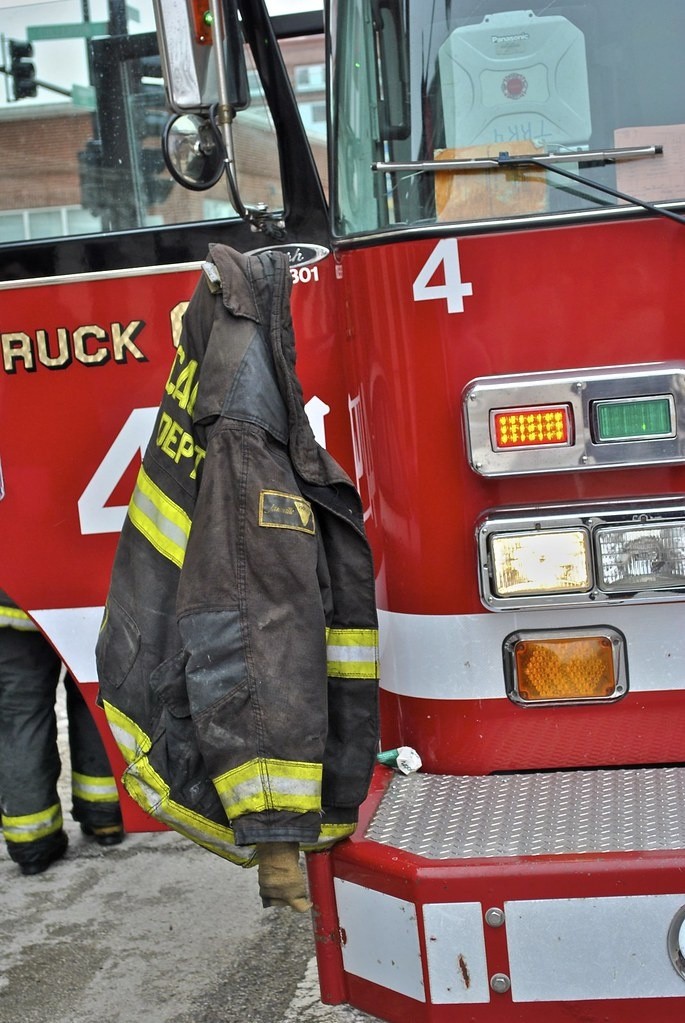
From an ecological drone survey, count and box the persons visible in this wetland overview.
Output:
[0,588,125,875]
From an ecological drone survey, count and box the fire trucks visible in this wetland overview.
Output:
[1,0,685,1023]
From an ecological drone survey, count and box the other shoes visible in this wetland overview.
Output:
[19,831,68,875]
[80,821,122,845]
